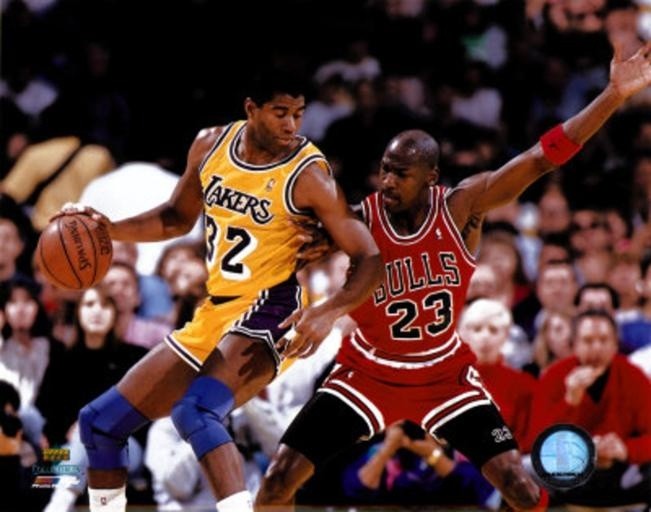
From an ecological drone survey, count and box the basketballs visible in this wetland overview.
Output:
[38,214,112,289]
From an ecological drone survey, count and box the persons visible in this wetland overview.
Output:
[1,0,651,510]
